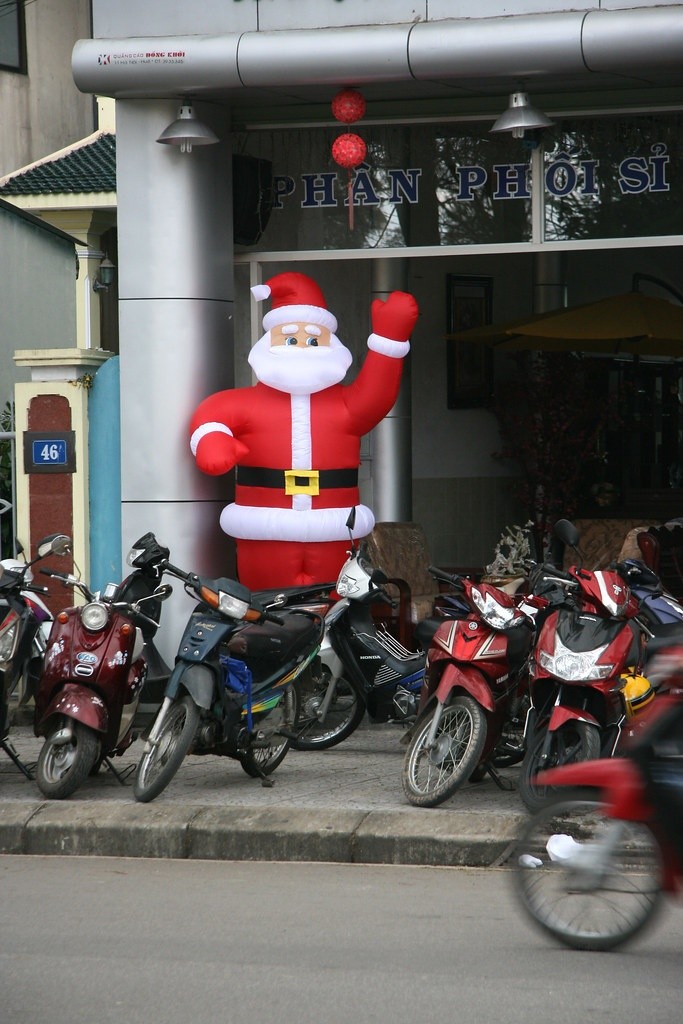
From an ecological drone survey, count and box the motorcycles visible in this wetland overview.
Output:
[0,515,683,958]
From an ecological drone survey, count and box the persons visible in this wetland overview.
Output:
[189,271,420,591]
